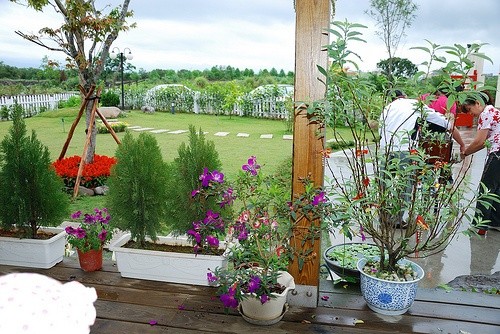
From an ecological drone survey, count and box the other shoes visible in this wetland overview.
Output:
[379,217,411,230]
[477,228,488,236]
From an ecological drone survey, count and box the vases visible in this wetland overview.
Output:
[357,257,424,316]
[239,267,296,319]
[77,247,102,272]
[324,243,387,282]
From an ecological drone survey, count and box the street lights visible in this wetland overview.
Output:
[109,47,133,107]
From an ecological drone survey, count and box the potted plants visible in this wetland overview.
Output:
[0,98,66,269]
[104,124,234,286]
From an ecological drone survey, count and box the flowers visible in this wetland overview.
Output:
[64,207,113,256]
[302,0,500,271]
[189,155,327,307]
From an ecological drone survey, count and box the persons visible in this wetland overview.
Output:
[378,89,465,229]
[459,92,500,235]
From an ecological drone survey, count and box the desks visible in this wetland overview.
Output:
[455,113,473,129]
